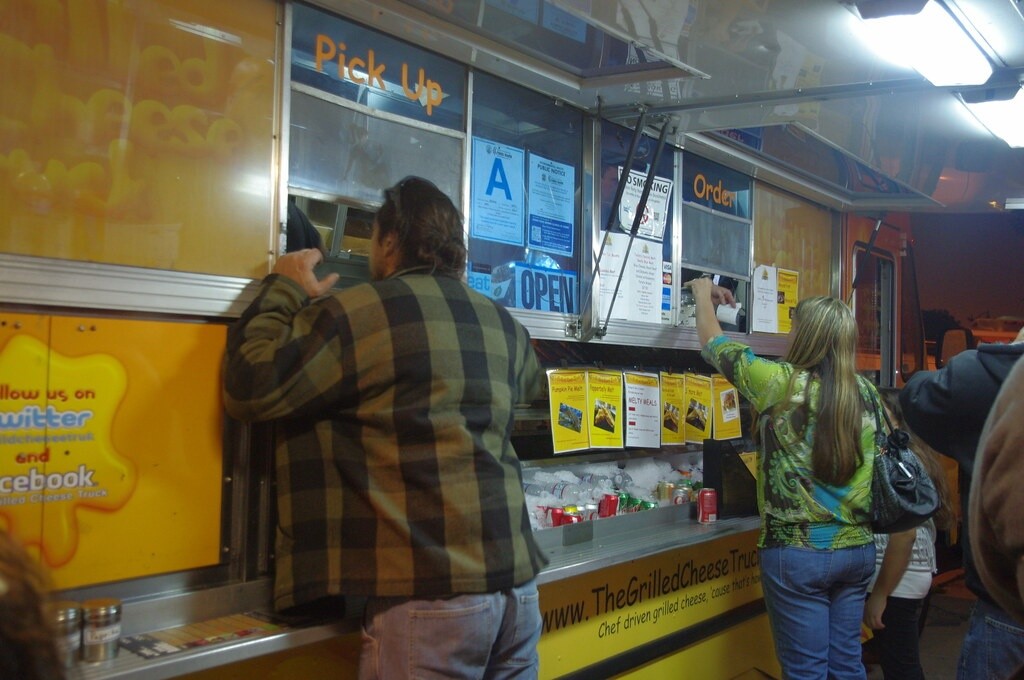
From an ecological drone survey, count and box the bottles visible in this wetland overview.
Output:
[523,471,634,531]
[83,599,121,660]
[47,601,82,668]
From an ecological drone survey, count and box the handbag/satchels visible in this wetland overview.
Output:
[860,375,940,534]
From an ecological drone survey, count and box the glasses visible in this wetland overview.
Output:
[389,181,402,219]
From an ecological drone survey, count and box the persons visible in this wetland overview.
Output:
[221,175,550,680]
[600,157,633,232]
[286,198,329,257]
[684,277,954,680]
[898,323,1024,680]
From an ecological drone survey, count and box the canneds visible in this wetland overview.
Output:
[698,487,717,525]
[533,478,702,527]
